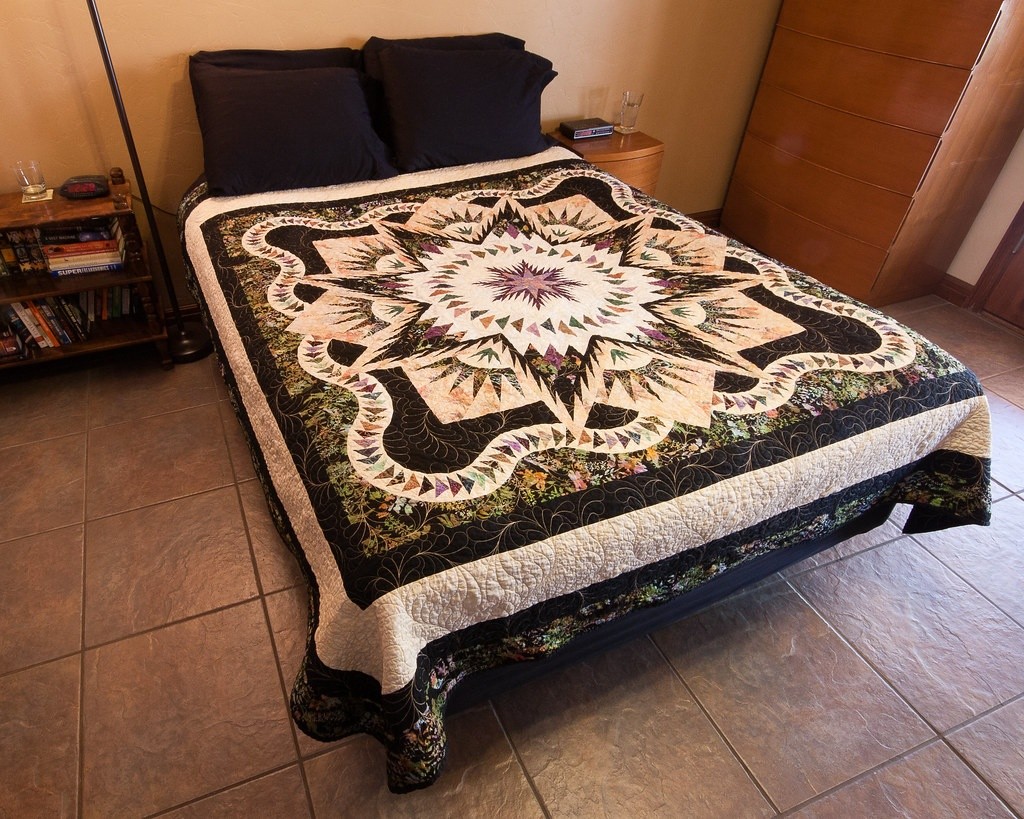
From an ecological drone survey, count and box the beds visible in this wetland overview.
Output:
[172,131,991,798]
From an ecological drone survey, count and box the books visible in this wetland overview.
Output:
[1,215,163,358]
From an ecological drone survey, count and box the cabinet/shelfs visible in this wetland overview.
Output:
[717,0,1024,309]
[0,167,173,383]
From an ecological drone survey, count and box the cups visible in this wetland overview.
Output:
[12,160,48,199]
[620,91,645,132]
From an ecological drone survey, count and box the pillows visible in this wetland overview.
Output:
[193,45,386,145]
[361,32,528,148]
[188,55,402,197]
[381,45,558,174]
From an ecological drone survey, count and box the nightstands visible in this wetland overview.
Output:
[545,123,665,200]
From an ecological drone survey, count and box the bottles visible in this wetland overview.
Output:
[108,167,132,210]
